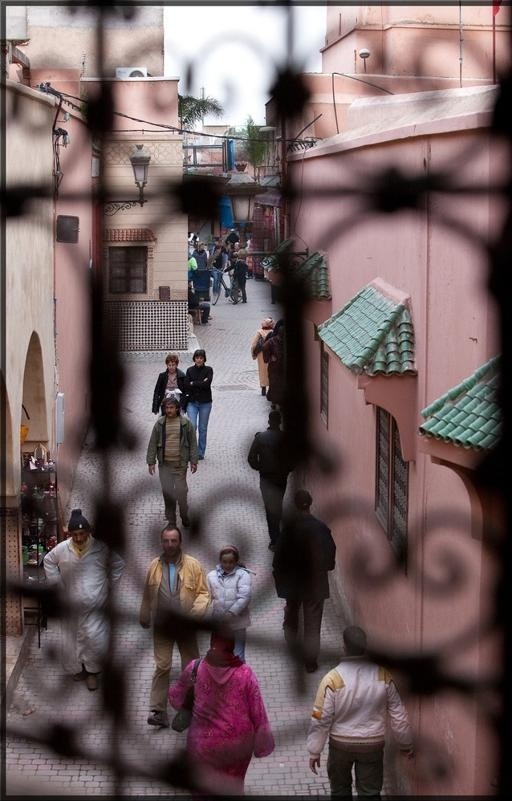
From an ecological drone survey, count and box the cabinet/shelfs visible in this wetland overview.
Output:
[21,450,62,648]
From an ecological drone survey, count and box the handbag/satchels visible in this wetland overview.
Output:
[253,332,264,356]
[169,658,200,735]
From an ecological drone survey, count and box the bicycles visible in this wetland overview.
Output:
[212,267,243,306]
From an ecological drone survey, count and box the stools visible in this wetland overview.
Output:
[188,308,204,325]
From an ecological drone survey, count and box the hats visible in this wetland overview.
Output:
[68,508,90,532]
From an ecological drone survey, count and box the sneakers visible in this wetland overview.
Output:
[198,455,205,460]
[234,300,247,304]
[201,318,209,324]
[304,657,319,675]
[267,541,279,552]
[67,670,99,692]
[181,517,191,527]
[261,387,267,395]
[147,711,171,729]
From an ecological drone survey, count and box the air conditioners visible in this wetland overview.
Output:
[116,65,150,79]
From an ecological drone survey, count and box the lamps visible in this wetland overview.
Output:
[95,142,153,217]
[357,46,371,74]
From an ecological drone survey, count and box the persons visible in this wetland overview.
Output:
[184,350,213,460]
[152,355,188,419]
[206,546,251,663]
[273,490,337,671]
[188,228,247,326]
[248,411,294,551]
[139,529,211,728]
[251,317,284,410]
[147,398,198,527]
[169,627,276,801]
[308,627,415,801]
[44,515,126,690]
